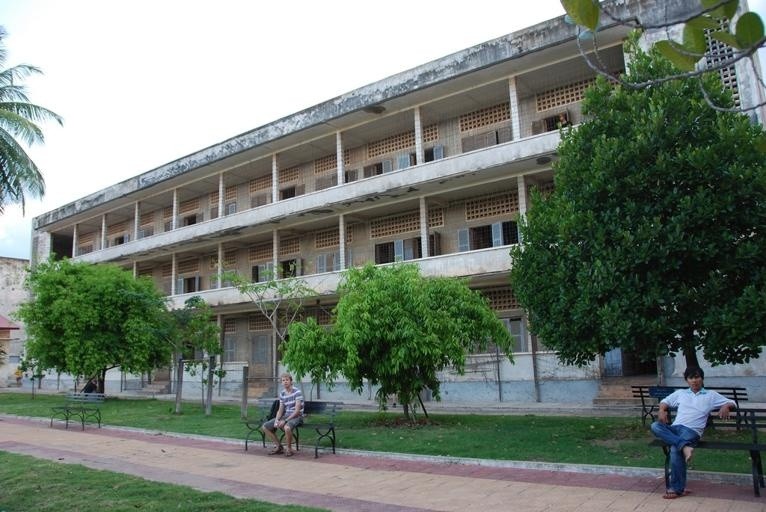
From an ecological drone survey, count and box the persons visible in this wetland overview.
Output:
[84,379,97,396]
[650,365,737,499]
[555,111,572,130]
[262,374,304,456]
[15,365,23,386]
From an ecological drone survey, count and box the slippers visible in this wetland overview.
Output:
[662,490,678,499]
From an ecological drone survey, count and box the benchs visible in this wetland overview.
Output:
[240,400,344,458]
[631,386,766,497]
[47,393,106,431]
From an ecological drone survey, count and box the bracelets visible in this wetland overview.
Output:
[284,418,287,423]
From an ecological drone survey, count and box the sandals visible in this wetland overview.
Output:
[272,445,283,455]
[286,452,293,457]
